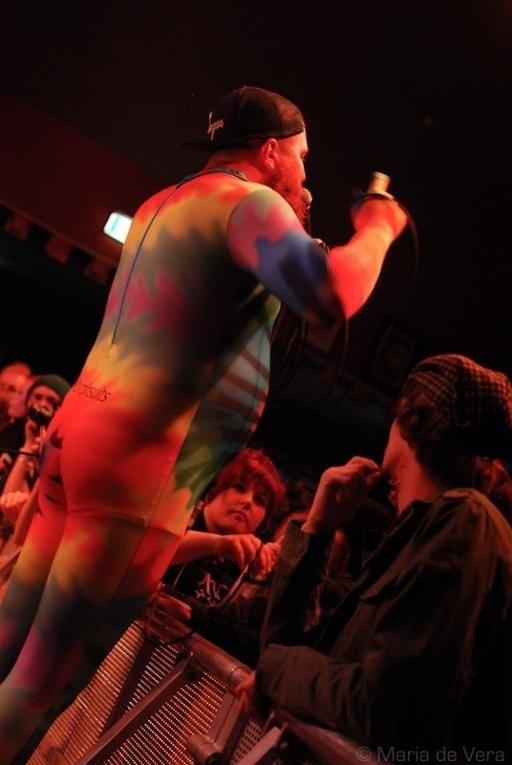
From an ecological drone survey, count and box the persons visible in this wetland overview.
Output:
[0,84,411,765]
[2,355,511,765]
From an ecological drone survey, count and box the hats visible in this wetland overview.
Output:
[404,352,511,443]
[178,85,305,148]
[25,373,70,407]
